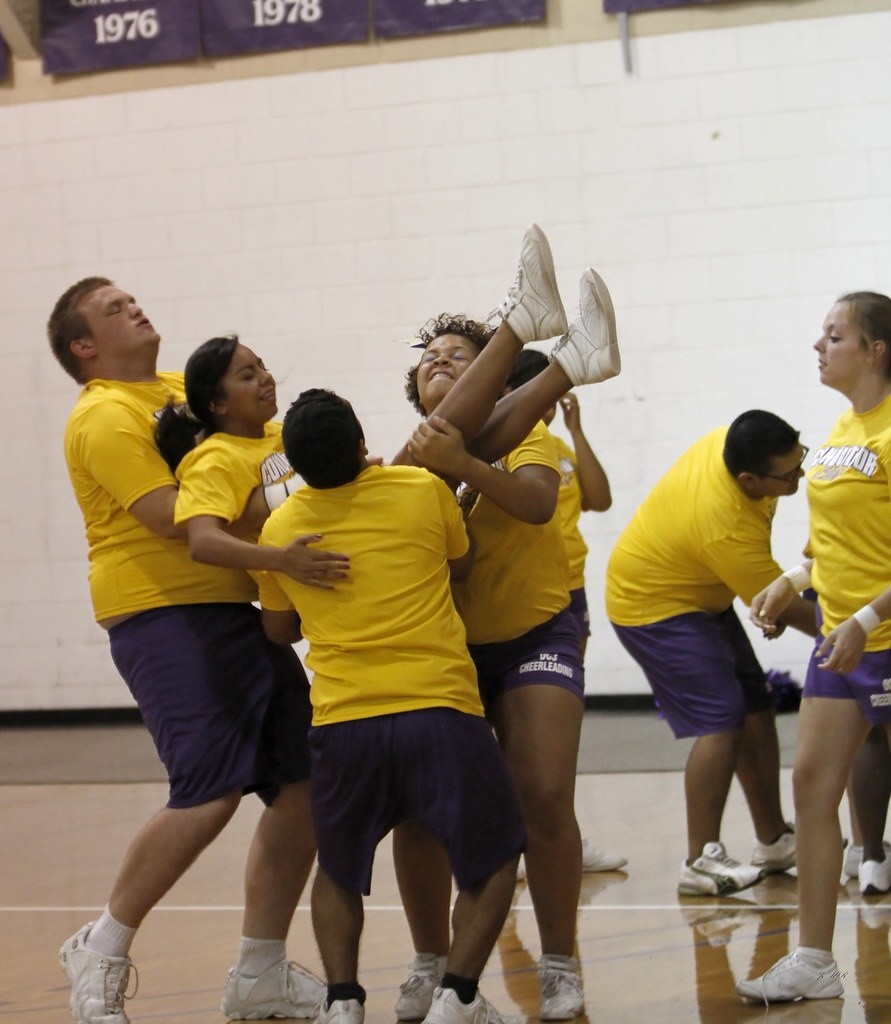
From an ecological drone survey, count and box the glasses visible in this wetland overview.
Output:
[767,444,810,483]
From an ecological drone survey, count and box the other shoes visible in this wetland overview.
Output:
[495,881,526,938]
[676,841,766,896]
[843,838,891,877]
[676,896,759,947]
[750,820,849,875]
[860,894,891,930]
[581,836,628,872]
[858,846,891,896]
[579,870,628,906]
[752,871,851,907]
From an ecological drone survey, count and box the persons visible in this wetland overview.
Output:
[48,225,632,1023]
[737,292,891,1000]
[606,409,816,896]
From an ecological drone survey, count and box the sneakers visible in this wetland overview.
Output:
[538,940,586,1020]
[219,957,325,1018]
[486,222,570,344]
[733,951,845,1008]
[393,951,448,1021]
[307,993,366,1024]
[58,921,140,1024]
[421,987,508,1024]
[547,266,622,386]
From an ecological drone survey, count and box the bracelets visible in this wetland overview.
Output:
[782,564,811,594]
[851,605,879,634]
